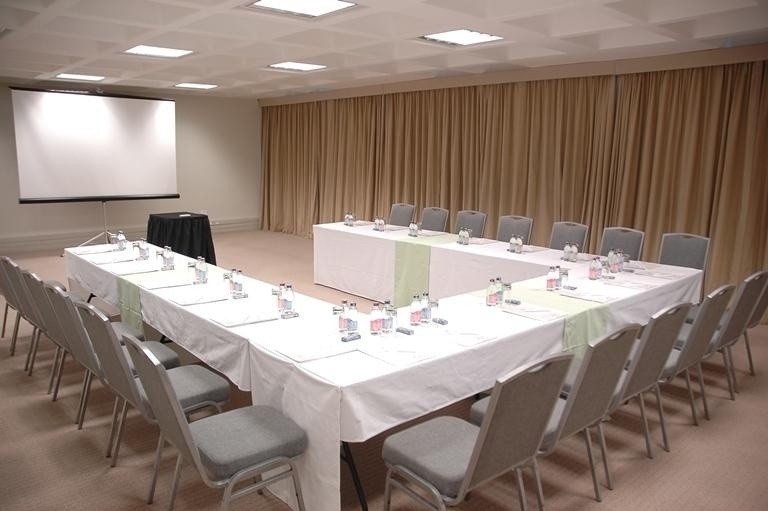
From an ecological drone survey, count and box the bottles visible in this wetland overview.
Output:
[117,231,126,250]
[338,301,358,336]
[563,241,577,262]
[588,248,624,281]
[344,212,354,226]
[410,292,431,325]
[373,216,385,232]
[194,257,206,280]
[228,268,243,293]
[370,299,393,335]
[408,221,418,236]
[161,246,173,265]
[486,276,504,307]
[508,234,522,252]
[277,285,292,311]
[458,227,469,245]
[138,238,150,258]
[544,265,562,292]
